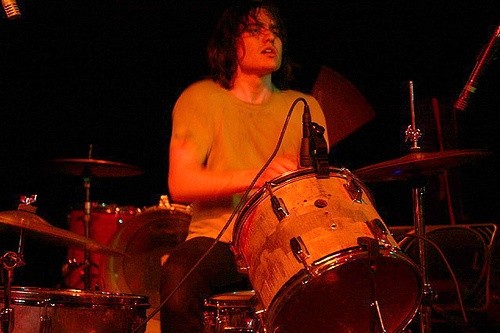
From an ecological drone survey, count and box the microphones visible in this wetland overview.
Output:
[454,26,500,111]
[0,0,22,21]
[300,105,313,166]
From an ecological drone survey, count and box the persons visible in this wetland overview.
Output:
[160,0,330,333]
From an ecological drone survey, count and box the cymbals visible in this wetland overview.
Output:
[353,149,493,182]
[55,159,139,178]
[0,211,131,258]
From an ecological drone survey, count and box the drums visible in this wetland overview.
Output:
[203,291,258,330]
[65,207,160,333]
[232,167,424,333]
[111,205,193,320]
[0,286,149,333]
